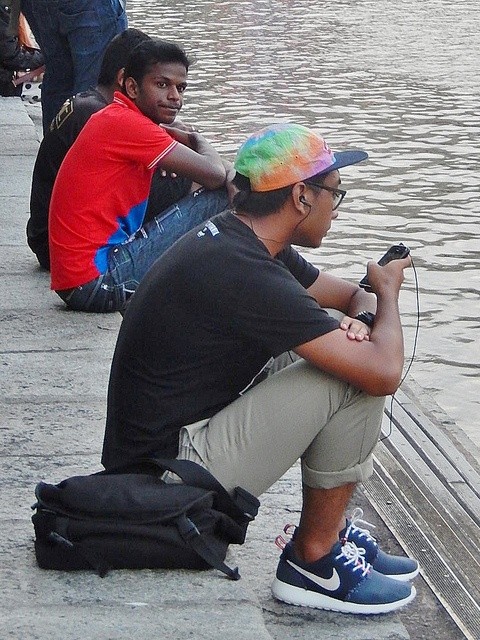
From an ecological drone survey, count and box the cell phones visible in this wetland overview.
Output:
[358,244,410,289]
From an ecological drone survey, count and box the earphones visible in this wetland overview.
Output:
[233,193,422,443]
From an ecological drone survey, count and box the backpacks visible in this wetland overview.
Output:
[31,457,262,579]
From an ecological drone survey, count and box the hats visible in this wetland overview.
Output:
[227,124,368,193]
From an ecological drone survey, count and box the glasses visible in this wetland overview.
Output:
[309,181,346,211]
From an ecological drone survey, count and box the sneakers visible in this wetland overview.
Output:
[284,507,419,582]
[272,536,416,613]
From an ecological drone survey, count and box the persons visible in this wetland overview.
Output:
[48,38,240,313]
[101,123,421,614]
[27,29,195,271]
[20,0,126,140]
